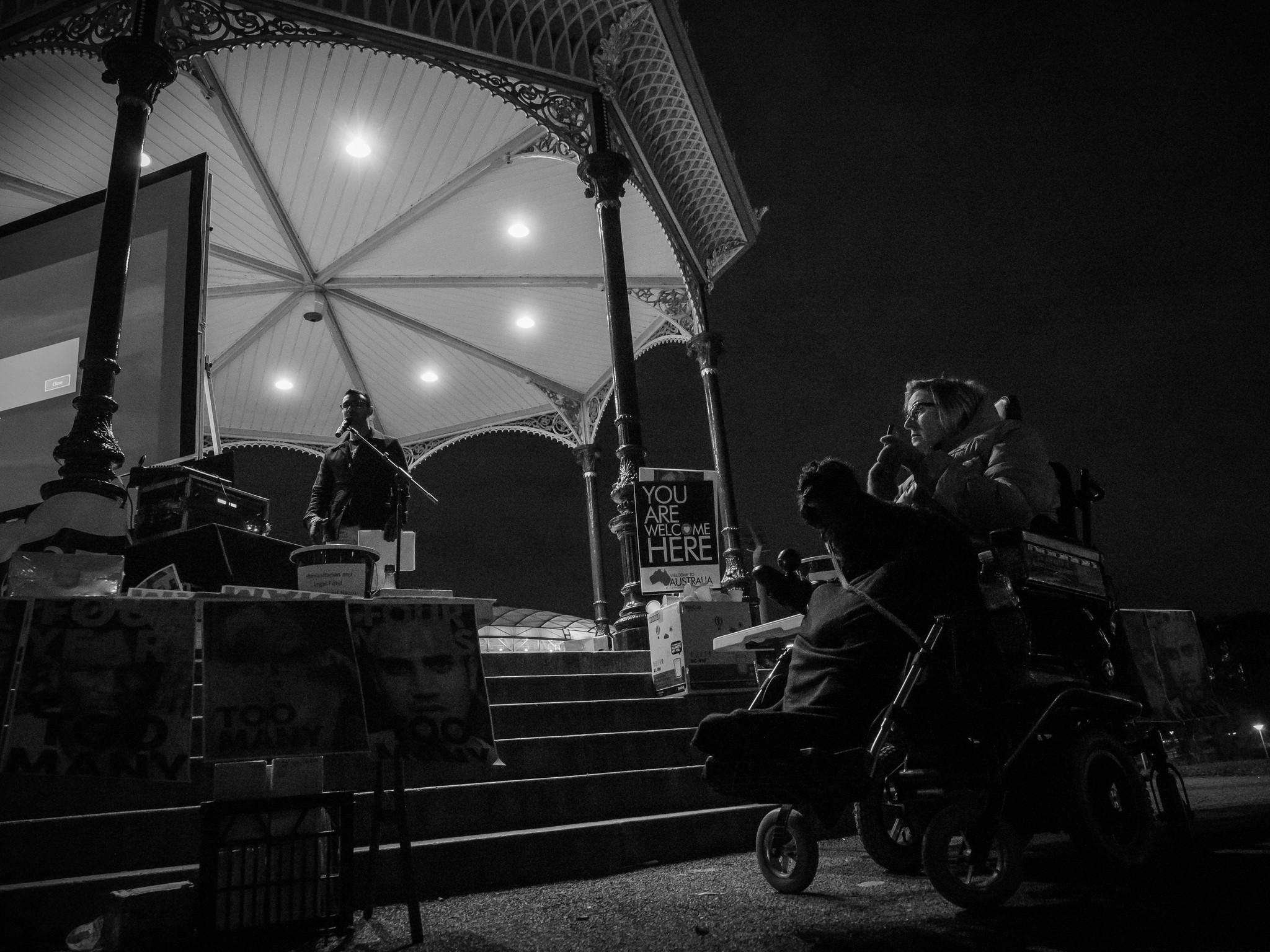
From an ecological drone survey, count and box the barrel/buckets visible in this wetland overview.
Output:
[289,543,381,599]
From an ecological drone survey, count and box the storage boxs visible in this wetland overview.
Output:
[1020,530,1108,602]
[134,473,270,545]
[6,552,125,598]
[647,602,760,698]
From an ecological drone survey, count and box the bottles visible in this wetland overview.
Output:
[382,563,397,589]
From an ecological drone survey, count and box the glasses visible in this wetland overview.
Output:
[908,402,935,418]
[339,401,368,409]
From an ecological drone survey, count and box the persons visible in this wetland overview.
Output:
[1147,613,1227,719]
[302,388,411,545]
[690,377,1063,801]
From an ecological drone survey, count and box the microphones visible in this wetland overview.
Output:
[335,417,353,438]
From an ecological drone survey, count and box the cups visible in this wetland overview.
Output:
[645,583,743,614]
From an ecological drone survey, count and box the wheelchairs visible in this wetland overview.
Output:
[702,464,1205,908]
